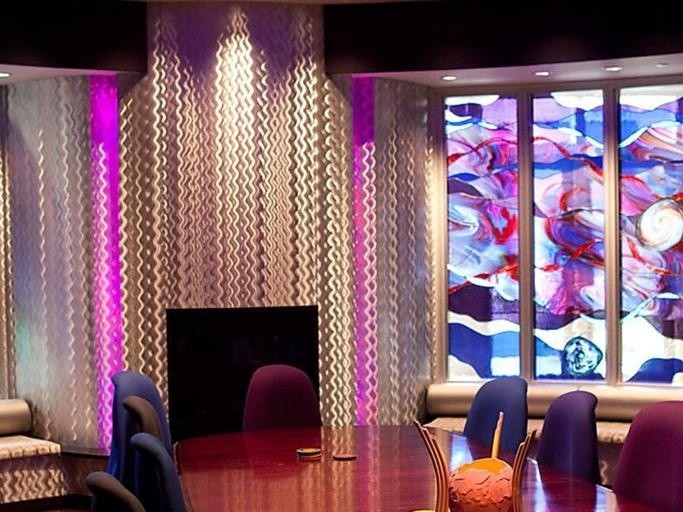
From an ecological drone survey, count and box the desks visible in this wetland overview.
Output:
[176,425,674,512]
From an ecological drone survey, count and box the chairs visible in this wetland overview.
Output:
[242,364,323,431]
[85,370,188,512]
[463,376,683,512]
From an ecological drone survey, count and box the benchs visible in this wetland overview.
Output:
[418,382,682,488]
[0,398,68,503]
